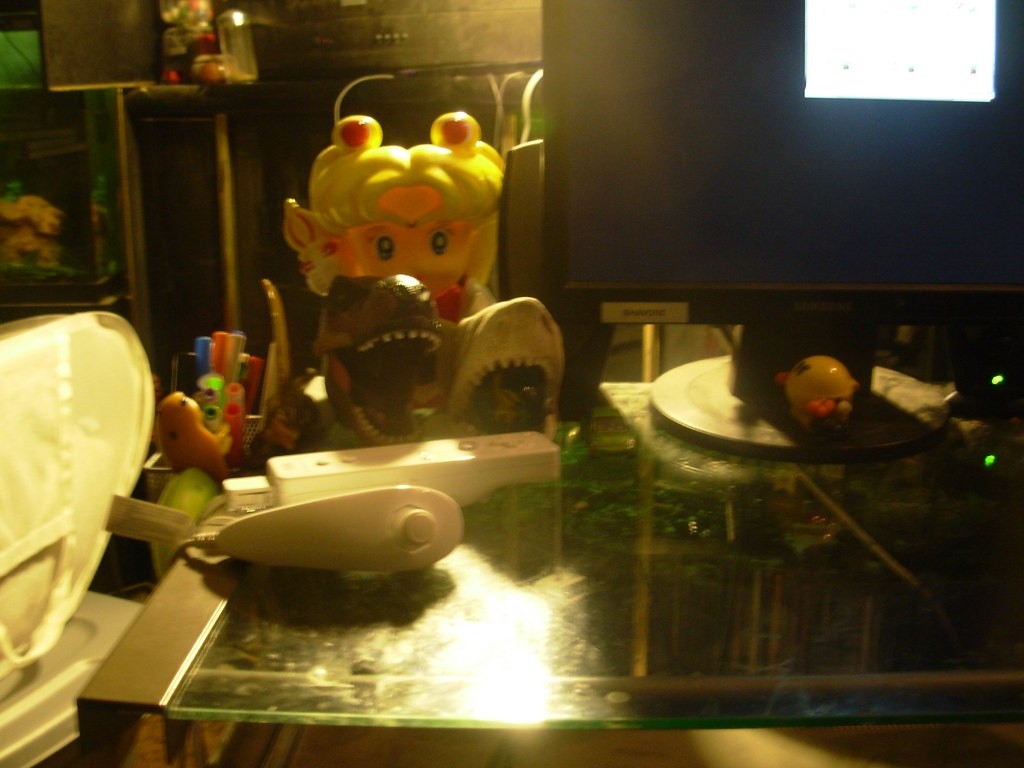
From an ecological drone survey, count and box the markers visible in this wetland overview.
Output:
[170,328,267,468]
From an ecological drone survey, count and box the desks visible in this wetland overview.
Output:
[75,381,1023,763]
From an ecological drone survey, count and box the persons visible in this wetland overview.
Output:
[284,113,507,323]
